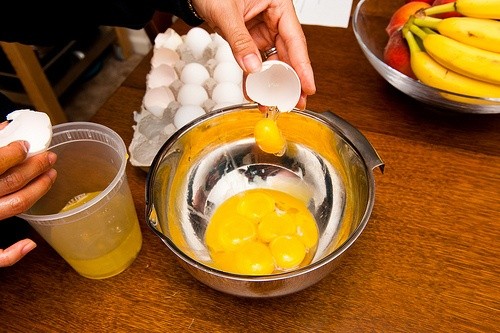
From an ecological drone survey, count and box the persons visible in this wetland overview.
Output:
[0,0,319,268]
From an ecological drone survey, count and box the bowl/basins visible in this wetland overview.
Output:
[145,102,385,299]
[352,0,500,114]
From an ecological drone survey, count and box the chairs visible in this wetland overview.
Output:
[1,26,135,126]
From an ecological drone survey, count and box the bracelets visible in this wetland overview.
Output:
[186,0,204,23]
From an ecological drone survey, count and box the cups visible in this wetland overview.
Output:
[16,122,143,279]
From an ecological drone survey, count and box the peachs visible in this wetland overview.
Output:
[385,0,463,78]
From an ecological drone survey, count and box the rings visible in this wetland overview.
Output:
[263,46,278,59]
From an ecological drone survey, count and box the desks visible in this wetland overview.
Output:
[0,19,500,333]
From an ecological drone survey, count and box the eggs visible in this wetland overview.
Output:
[254,107,287,157]
[204,191,319,275]
[144,27,245,131]
[45,190,142,282]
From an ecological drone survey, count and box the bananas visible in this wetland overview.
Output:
[400,0,500,105]
